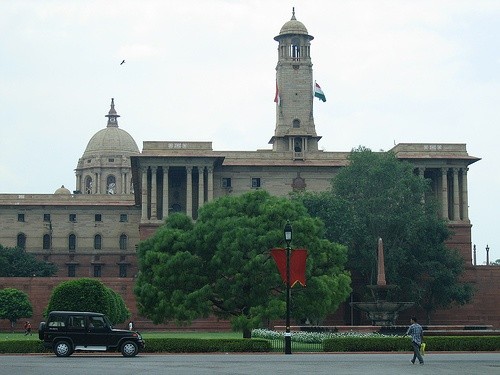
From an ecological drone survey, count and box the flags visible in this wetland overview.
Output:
[274,81,279,103]
[315,83,326,102]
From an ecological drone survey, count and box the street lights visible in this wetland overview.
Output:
[485,245,490,265]
[283,219,293,355]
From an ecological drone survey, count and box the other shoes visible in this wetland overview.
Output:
[419,362,424,366]
[410,360,414,365]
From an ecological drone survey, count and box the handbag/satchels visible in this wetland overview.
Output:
[420,342,426,356]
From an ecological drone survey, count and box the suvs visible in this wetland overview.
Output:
[38,310,145,358]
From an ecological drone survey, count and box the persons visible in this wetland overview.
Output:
[90,319,94,327]
[24,321,32,336]
[129,321,135,330]
[403,317,424,365]
